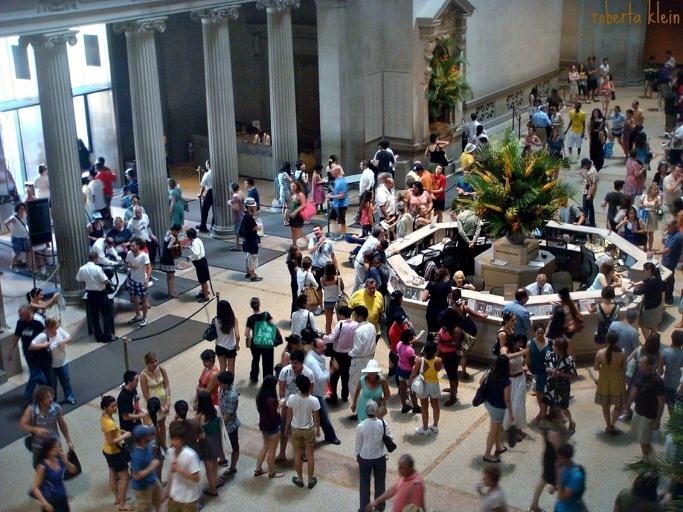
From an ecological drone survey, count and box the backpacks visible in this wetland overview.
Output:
[598,304,618,338]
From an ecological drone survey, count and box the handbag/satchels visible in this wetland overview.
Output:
[637,231,647,244]
[323,344,333,357]
[456,331,477,357]
[25,435,35,451]
[35,347,52,371]
[438,151,449,166]
[294,172,306,190]
[411,375,423,395]
[253,321,278,348]
[203,325,217,340]
[65,449,81,480]
[301,284,322,307]
[335,295,349,308]
[300,202,317,220]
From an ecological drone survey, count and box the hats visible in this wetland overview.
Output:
[244,197,257,207]
[401,504,424,512]
[134,424,155,438]
[580,158,588,166]
[361,360,382,373]
[92,212,102,219]
[464,143,476,153]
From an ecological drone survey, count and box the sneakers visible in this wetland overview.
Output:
[231,247,242,251]
[197,292,210,302]
[246,272,263,281]
[128,313,148,327]
[169,291,180,298]
[584,99,600,104]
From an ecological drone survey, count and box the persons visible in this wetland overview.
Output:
[0,47,682,511]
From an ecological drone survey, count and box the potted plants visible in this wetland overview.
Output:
[451,126,579,244]
[427,35,472,138]
